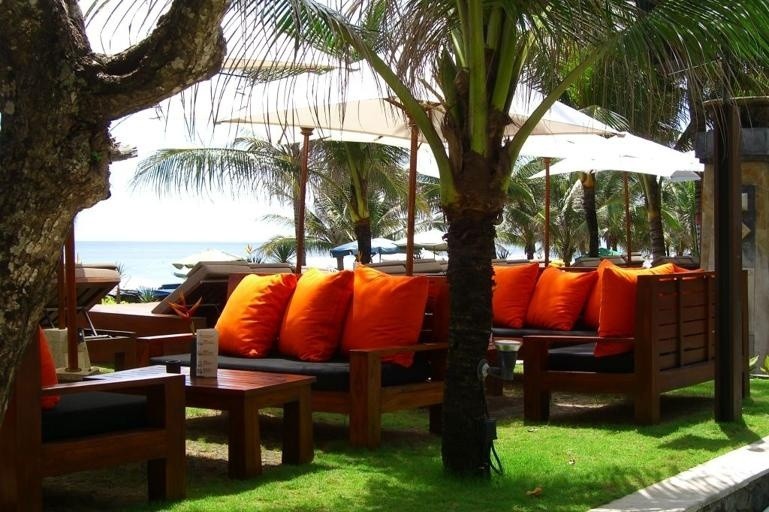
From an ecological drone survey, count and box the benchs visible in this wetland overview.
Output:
[519,264,753,428]
[2,320,188,512]
[479,258,684,348]
[117,258,449,447]
[89,259,300,340]
[41,259,122,330]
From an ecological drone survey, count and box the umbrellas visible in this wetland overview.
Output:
[64,0,360,372]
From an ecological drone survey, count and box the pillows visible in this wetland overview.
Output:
[213,273,295,356]
[338,255,430,370]
[33,320,62,408]
[487,255,708,357]
[277,265,355,363]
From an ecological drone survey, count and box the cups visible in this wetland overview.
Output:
[166,359,180,373]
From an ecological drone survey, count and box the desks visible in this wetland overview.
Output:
[85,362,315,480]
